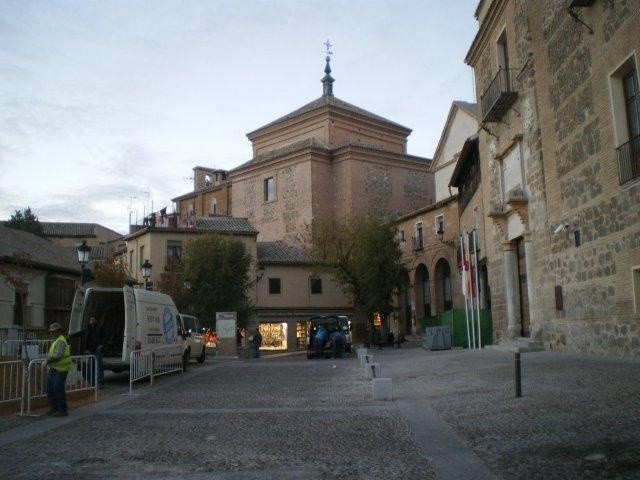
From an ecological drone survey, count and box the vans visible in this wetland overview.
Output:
[306,315,353,359]
[180,314,206,363]
[67,286,192,387]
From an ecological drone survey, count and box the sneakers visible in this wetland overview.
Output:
[54,411,68,416]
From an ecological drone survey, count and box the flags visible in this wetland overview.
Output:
[460,235,477,298]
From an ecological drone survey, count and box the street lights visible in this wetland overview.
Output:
[142,259,152,290]
[77,240,90,286]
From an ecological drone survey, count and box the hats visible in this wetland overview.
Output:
[48,322,63,331]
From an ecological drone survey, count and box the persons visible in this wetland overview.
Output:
[315,327,345,359]
[365,330,404,350]
[84,314,105,389]
[252,327,261,358]
[43,322,72,416]
